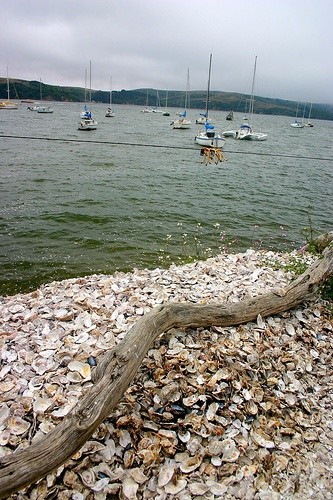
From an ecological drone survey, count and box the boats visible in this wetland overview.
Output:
[225,107,234,120]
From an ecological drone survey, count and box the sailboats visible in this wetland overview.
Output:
[76,59,98,132]
[290,100,315,128]
[139,65,216,130]
[0,66,55,114]
[104,75,115,117]
[243,99,249,120]
[193,53,228,149]
[222,55,268,142]
